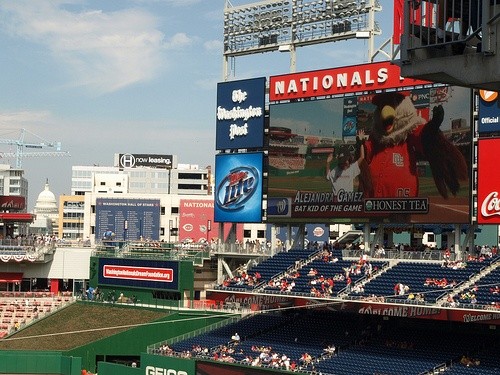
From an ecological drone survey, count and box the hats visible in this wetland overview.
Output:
[337,150,350,157]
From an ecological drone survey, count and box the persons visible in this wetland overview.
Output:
[0,233,500,375]
[323,89,468,225]
